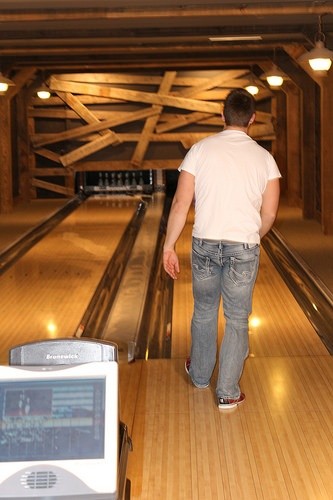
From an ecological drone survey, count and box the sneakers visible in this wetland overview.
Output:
[217,392,247,410]
[184,355,210,389]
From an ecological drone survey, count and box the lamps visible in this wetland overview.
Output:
[0,72,16,92]
[243,76,260,95]
[297,14,333,71]
[258,47,287,86]
[34,82,52,100]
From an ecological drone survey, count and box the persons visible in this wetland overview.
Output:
[162,87,281,408]
